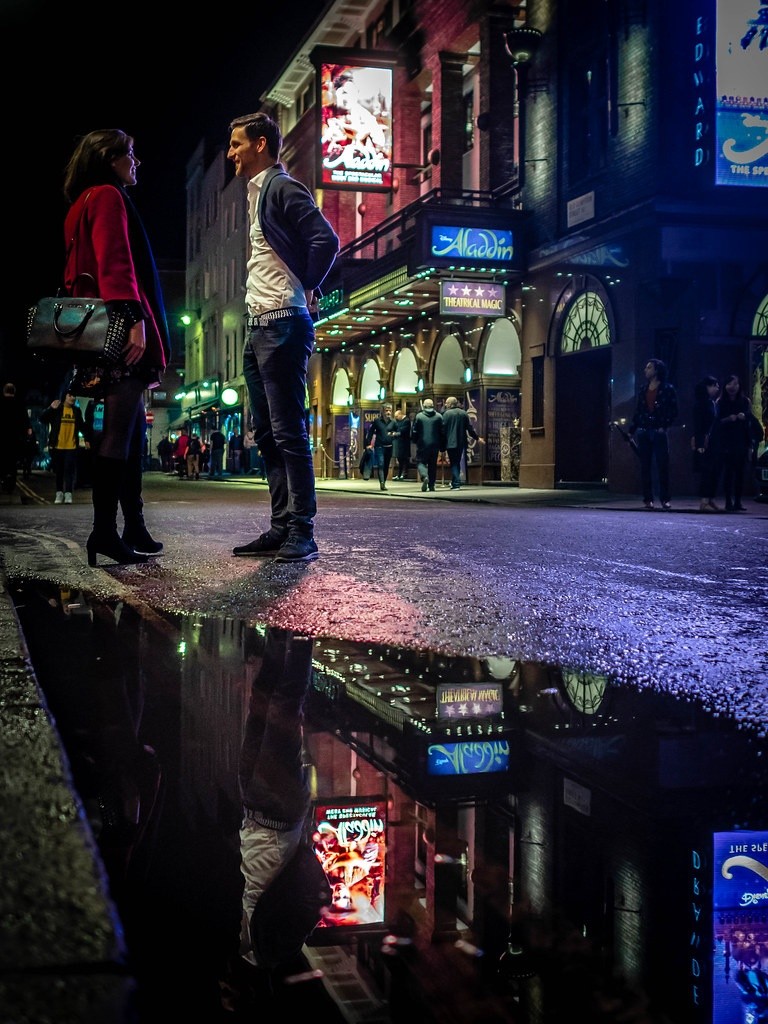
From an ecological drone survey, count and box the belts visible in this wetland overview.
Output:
[244,307,310,327]
[241,805,307,832]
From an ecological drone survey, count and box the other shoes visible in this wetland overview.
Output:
[645,504,654,509]
[663,504,671,509]
[725,503,737,511]
[709,502,726,512]
[63,492,73,504]
[700,503,715,512]
[165,468,241,482]
[54,491,64,505]
[378,469,462,493]
[734,504,747,511]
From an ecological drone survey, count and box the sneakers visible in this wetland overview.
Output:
[273,535,319,562]
[233,531,285,557]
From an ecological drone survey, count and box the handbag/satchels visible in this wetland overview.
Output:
[746,410,765,442]
[26,272,128,367]
[691,435,709,450]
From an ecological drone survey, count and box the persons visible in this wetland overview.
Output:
[82,592,163,833]
[365,401,402,490]
[0,380,104,505]
[719,376,751,512]
[694,374,722,513]
[244,425,258,448]
[391,409,411,481]
[225,112,340,564]
[441,395,486,490]
[411,397,443,492]
[626,358,681,509]
[228,425,243,476]
[157,426,225,482]
[228,628,334,991]
[62,128,164,566]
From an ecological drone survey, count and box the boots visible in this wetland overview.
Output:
[86,455,149,566]
[119,455,162,553]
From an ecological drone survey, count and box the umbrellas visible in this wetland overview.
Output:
[608,424,645,469]
